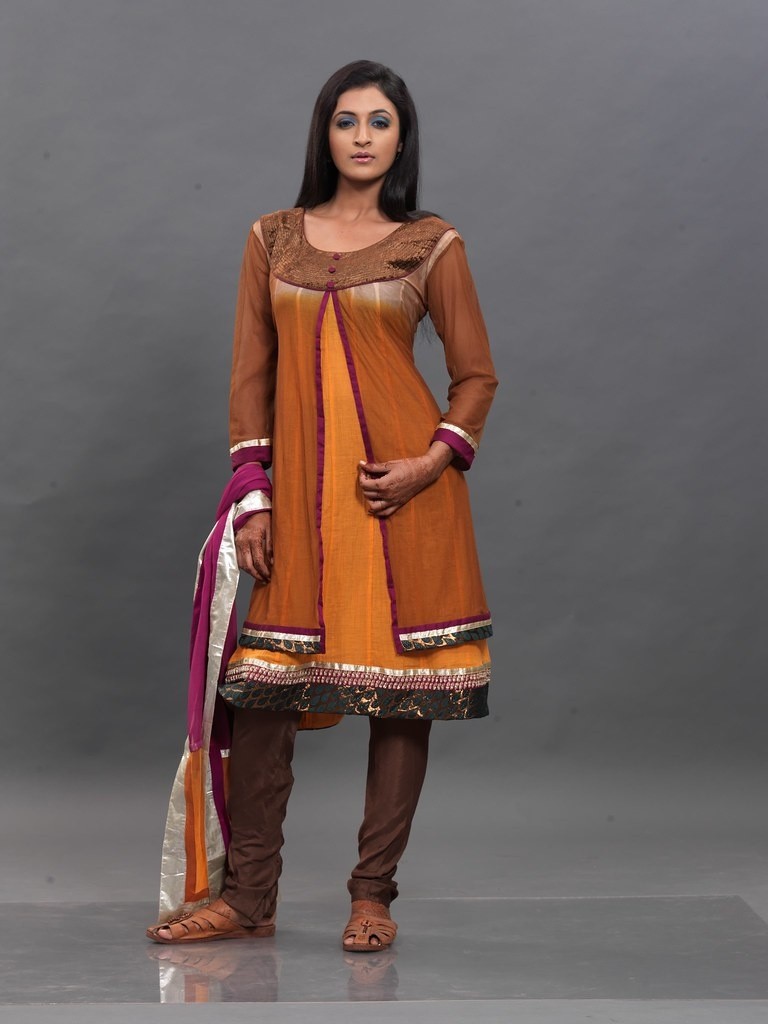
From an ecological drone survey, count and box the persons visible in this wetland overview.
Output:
[146,60,497,954]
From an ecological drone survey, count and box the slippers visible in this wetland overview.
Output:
[342,912,398,951]
[146,907,276,944]
